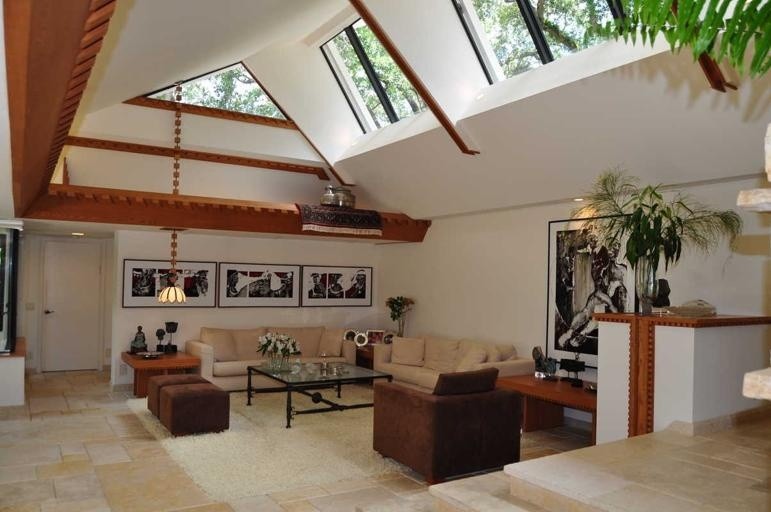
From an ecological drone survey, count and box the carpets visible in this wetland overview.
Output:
[125,389,417,501]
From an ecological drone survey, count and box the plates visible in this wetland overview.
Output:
[354,334,369,346]
[384,334,394,344]
[138,351,165,358]
[345,329,357,342]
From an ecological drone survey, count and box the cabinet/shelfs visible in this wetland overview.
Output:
[122,348,200,396]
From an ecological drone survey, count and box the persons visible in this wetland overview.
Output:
[187,271,208,297]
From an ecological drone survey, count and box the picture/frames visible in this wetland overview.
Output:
[365,329,385,347]
[300,264,373,309]
[216,260,302,310]
[123,256,218,310]
[546,215,654,368]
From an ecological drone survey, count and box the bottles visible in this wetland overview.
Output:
[319,186,355,208]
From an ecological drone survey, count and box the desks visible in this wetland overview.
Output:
[502,373,595,441]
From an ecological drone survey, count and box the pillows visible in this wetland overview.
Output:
[437,369,497,392]
[456,345,488,371]
[391,335,424,365]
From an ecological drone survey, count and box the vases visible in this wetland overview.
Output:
[268,350,283,370]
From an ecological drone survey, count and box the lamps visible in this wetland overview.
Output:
[158,83,187,304]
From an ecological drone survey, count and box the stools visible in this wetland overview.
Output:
[146,373,210,423]
[158,382,231,435]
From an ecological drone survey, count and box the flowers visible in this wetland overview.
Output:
[387,291,417,321]
[257,330,302,355]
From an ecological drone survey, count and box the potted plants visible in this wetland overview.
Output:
[586,172,743,313]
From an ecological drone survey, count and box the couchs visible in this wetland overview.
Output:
[372,378,522,479]
[371,335,538,392]
[188,327,357,391]
